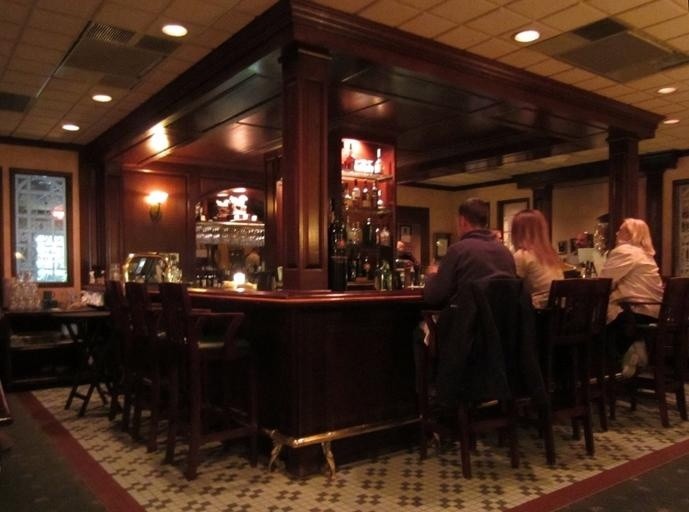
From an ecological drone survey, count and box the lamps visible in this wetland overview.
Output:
[224,272,257,292]
[145,192,167,220]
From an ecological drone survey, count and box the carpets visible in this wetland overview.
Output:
[19,383,689,512]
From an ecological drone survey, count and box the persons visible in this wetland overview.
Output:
[568,229,594,265]
[397,240,416,263]
[510,207,567,312]
[423,196,516,420]
[597,215,664,378]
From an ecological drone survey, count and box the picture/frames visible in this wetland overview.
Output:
[672,178,689,277]
[433,232,450,259]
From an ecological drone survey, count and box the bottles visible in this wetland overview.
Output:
[192,266,222,288]
[329,143,416,292]
[89,265,105,286]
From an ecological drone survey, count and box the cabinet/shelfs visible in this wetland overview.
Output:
[2,308,99,391]
[330,135,398,267]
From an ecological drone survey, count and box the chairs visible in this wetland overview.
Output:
[100,282,257,479]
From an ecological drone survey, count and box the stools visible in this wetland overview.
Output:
[50,308,111,414]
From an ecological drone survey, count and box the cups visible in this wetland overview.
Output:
[8,271,40,311]
[108,263,121,281]
